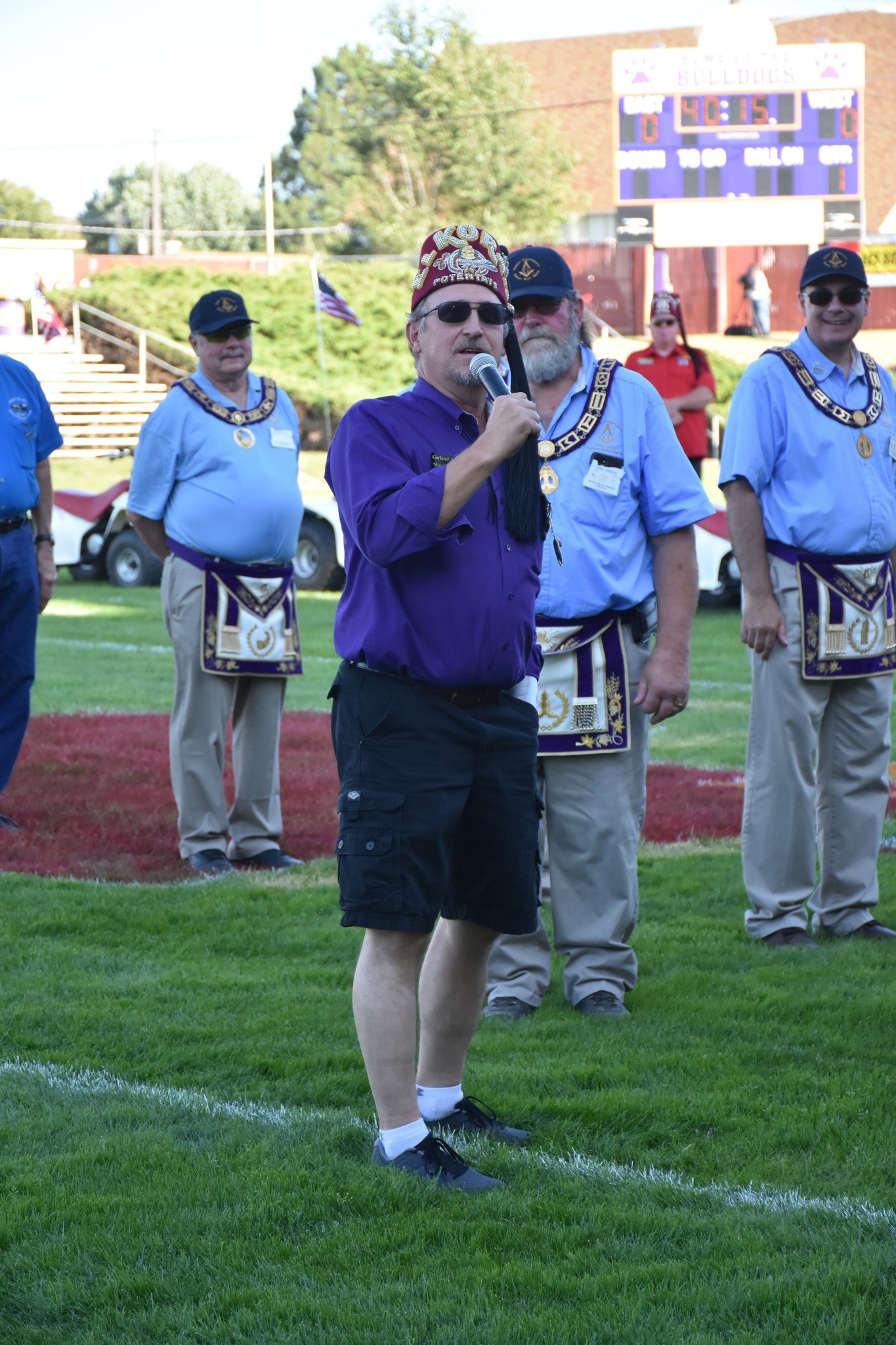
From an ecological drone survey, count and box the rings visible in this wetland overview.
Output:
[675,703,683,708]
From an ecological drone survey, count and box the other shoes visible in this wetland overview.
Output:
[187,848,233,873]
[853,918,896,939]
[762,929,822,951]
[251,848,305,869]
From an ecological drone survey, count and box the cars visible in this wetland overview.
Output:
[692,457,743,589]
[48,446,346,591]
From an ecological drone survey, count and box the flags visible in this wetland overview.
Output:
[314,270,362,326]
[33,286,69,343]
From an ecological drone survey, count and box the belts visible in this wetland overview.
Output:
[1,511,32,535]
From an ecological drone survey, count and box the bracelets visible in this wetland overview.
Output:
[34,535,55,547]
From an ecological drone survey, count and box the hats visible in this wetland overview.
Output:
[507,244,574,298]
[410,224,552,541]
[800,247,868,290]
[651,290,700,376]
[188,289,260,333]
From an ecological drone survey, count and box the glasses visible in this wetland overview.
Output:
[510,294,559,318]
[655,319,676,327]
[414,302,512,325]
[198,323,252,343]
[803,285,865,305]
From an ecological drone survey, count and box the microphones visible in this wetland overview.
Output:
[469,353,511,401]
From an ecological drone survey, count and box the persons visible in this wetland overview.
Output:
[482,244,716,1018]
[0,354,63,828]
[746,262,772,335]
[323,226,565,1191]
[126,291,304,875]
[717,247,896,949]
[625,291,715,480]
[739,264,772,335]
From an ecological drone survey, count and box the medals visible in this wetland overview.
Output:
[231,411,255,448]
[853,410,873,458]
[538,440,559,494]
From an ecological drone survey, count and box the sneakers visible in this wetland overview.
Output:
[370,1131,505,1194]
[577,992,631,1022]
[427,1096,532,1142]
[484,997,534,1019]
